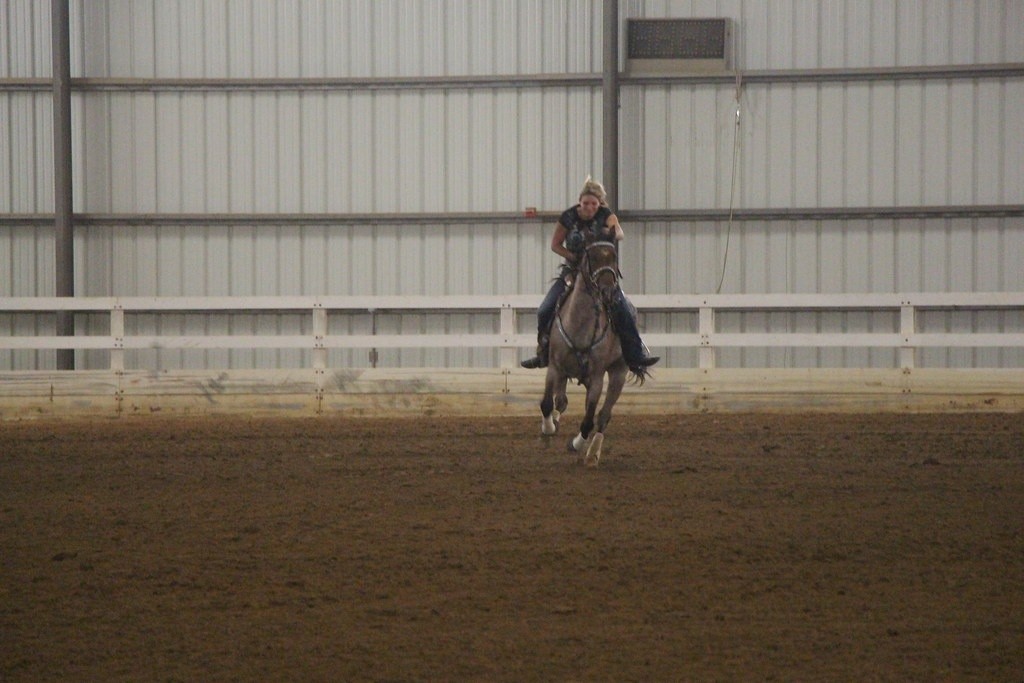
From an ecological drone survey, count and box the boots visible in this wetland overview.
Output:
[521,331,549,368]
[632,336,660,367]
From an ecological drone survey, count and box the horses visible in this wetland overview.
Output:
[540,225,656,472]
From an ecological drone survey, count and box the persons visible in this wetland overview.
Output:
[520,180,661,371]
